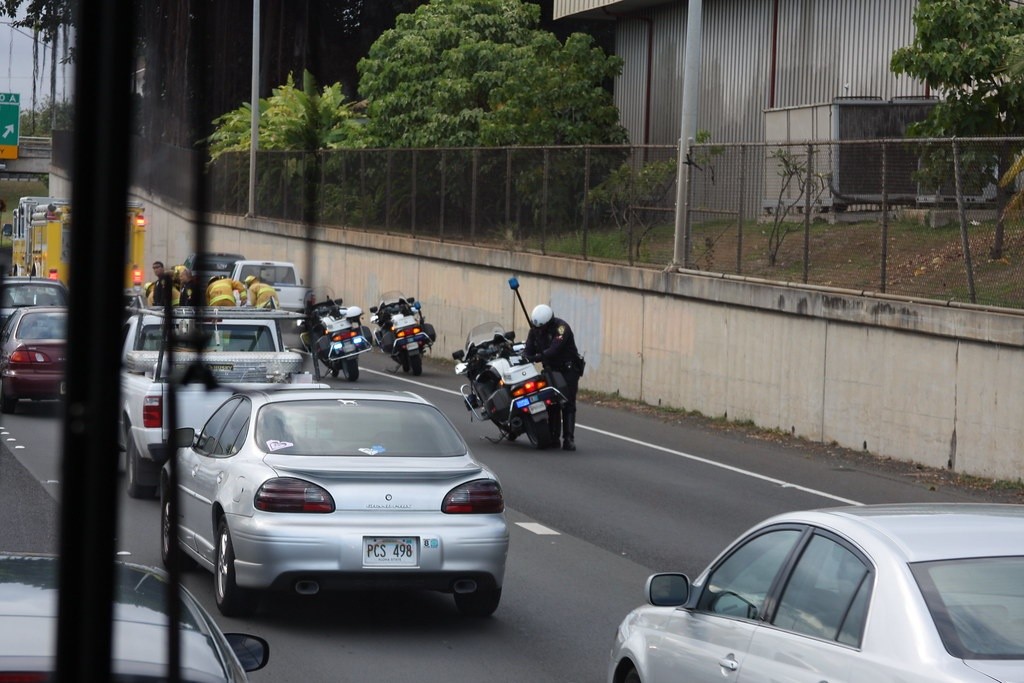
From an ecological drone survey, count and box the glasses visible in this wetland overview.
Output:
[153,266,161,270]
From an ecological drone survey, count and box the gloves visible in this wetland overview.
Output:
[535,354,542,361]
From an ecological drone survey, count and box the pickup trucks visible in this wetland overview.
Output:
[228,259,316,311]
[117,302,330,501]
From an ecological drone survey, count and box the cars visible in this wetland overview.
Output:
[157,386,512,619]
[607,502,1024,683]
[0,307,70,413]
[0,273,69,334]
[1,548,273,683]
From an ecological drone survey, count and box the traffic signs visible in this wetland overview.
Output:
[0,92,22,160]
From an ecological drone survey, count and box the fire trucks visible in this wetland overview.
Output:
[2,194,148,288]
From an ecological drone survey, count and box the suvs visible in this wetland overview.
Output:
[183,251,247,294]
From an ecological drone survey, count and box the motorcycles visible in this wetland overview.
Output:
[369,288,437,377]
[451,275,577,452]
[288,285,374,382]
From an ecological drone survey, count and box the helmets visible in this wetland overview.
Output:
[345,306,365,321]
[530,304,555,330]
[244,275,255,287]
[208,275,220,286]
[144,281,155,297]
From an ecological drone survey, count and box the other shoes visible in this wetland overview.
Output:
[552,438,562,448]
[563,437,577,450]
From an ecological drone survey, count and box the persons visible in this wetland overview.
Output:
[144,261,280,311]
[519,304,579,451]
